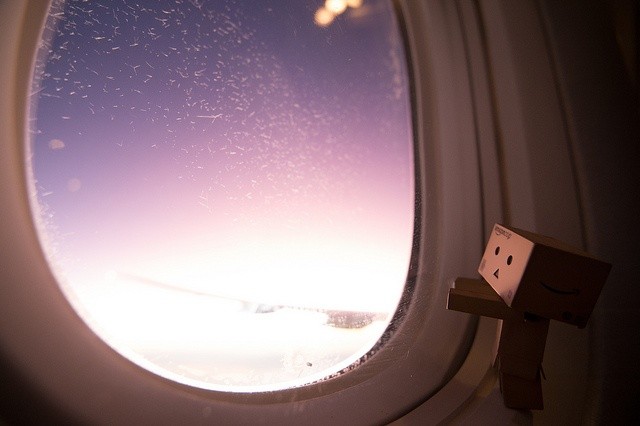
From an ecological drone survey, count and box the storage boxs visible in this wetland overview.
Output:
[477,219,614,329]
